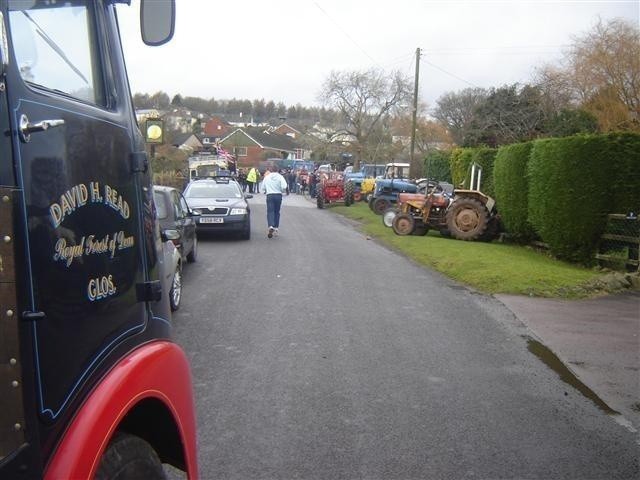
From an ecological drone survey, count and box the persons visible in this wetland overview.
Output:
[261,165,288,239]
[344,162,353,173]
[237,164,320,199]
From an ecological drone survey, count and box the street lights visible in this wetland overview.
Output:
[145,119,163,158]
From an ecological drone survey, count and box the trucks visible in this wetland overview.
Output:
[1,0,197,480]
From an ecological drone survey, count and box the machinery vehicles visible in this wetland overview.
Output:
[310,164,502,242]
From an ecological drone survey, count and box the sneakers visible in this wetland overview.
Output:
[267,226,279,238]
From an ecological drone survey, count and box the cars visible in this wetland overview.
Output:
[153,176,253,311]
